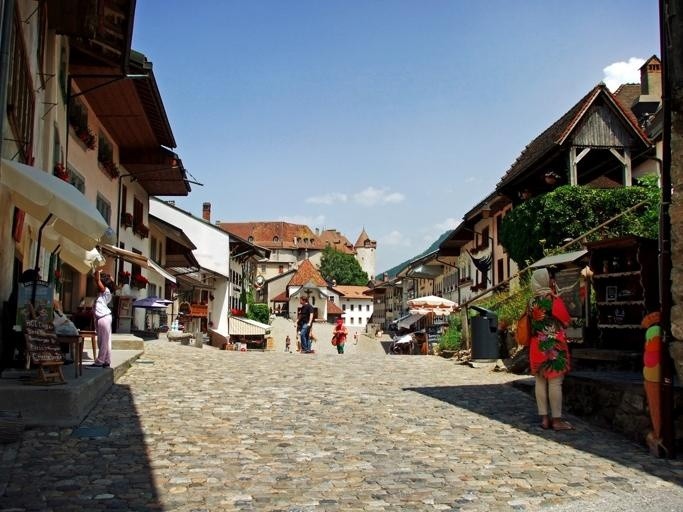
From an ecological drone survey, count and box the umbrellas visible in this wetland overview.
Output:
[406,306,453,327]
[132,298,168,331]
[404,294,459,324]
[146,295,174,305]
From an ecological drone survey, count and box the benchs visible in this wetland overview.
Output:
[13,330,97,378]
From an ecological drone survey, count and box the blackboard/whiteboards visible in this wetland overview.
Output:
[22,319,63,363]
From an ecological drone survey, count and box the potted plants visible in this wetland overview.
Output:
[135,274,148,289]
[119,271,130,284]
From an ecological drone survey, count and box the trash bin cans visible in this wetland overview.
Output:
[469,306,499,359]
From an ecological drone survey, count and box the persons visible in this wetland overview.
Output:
[285,335,290,351]
[296,295,314,354]
[354,331,358,345]
[295,336,300,352]
[3,269,74,365]
[332,317,348,355]
[294,311,303,352]
[85,268,114,369]
[522,267,576,432]
[415,328,429,355]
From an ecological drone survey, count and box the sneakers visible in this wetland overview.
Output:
[86,364,110,369]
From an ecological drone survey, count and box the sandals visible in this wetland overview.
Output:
[541,421,576,430]
